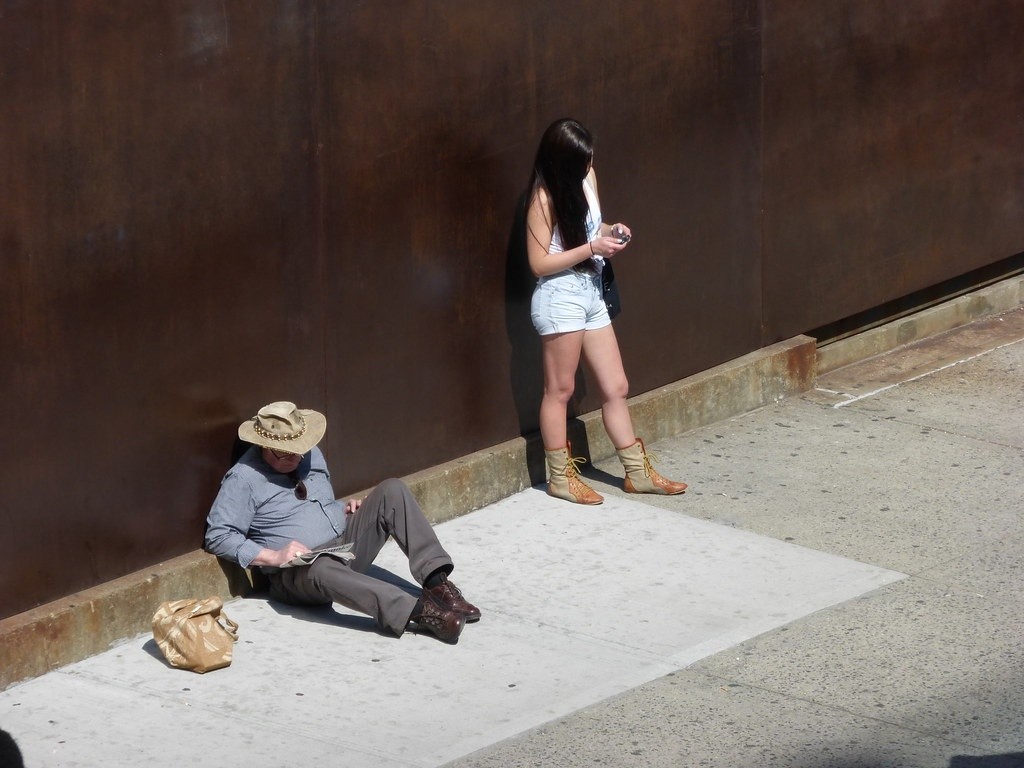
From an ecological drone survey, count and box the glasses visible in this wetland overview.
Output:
[290,468,307,500]
[270,449,296,461]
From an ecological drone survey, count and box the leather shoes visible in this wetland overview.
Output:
[423,571,481,622]
[410,596,467,642]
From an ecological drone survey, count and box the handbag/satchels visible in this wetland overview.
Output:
[152,595,239,674]
[599,257,621,320]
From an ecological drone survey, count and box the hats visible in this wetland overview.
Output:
[238,401,327,455]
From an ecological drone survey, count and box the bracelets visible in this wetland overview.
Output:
[590,241,595,256]
[610,224,615,238]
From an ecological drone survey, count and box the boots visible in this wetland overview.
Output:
[546,439,604,505]
[614,437,687,493]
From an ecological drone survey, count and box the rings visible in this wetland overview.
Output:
[296,551,303,556]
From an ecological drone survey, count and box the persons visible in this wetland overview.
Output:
[522,119,688,505]
[205,402,481,642]
